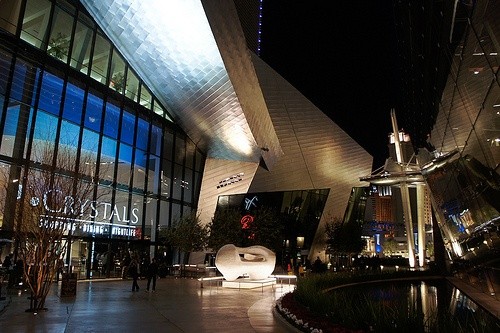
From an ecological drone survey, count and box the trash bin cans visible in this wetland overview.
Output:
[60,272,78,296]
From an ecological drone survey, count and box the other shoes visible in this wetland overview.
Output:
[132,289,135,291]
[137,287,139,289]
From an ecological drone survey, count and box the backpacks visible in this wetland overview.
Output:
[128,266,135,275]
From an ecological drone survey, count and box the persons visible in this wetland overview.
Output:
[127,259,140,292]
[94,249,149,278]
[16,257,24,287]
[146,258,160,293]
[55,259,64,283]
[315,256,322,273]
[1,254,14,285]
